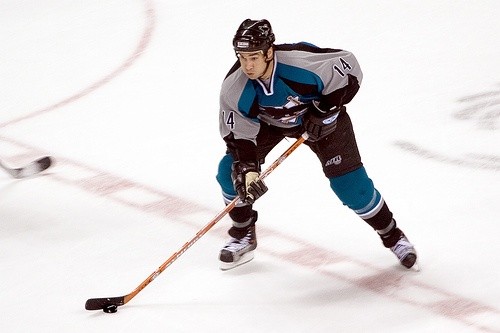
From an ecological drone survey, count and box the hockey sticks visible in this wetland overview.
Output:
[85,131,310,310]
[0,156,51,178]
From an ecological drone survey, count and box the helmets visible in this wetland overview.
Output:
[232,19,275,56]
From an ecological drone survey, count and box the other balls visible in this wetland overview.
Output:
[104,305,118,313]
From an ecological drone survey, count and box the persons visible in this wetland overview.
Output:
[216,18,421,272]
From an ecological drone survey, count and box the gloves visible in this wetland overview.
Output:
[231,160,268,205]
[296,100,341,143]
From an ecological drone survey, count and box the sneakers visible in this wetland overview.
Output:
[377,219,421,272]
[217,210,258,270]
